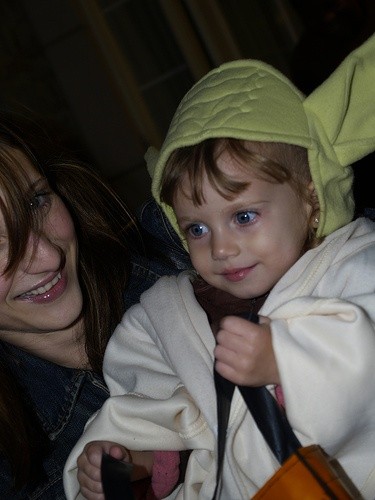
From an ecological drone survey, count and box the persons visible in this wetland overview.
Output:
[63,61,375,500]
[0,102,177,500]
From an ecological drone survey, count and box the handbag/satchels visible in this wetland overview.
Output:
[99,358,356,499]
